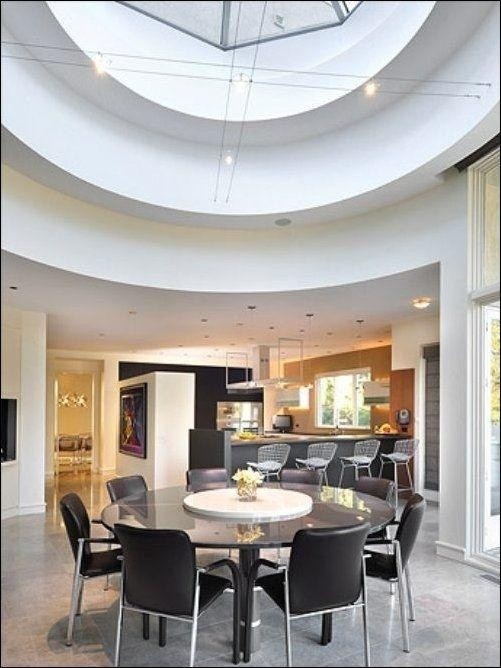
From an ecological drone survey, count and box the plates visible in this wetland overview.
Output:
[373,433,399,437]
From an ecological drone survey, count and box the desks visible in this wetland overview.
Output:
[100,481,396,654]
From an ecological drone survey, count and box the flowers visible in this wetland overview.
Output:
[232,466,265,487]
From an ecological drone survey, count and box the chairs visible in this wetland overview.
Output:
[104,474,149,591]
[114,523,241,668]
[60,493,149,646]
[58,435,92,457]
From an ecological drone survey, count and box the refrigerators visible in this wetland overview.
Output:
[216,401,262,435]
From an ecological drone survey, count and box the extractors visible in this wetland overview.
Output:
[225,339,311,390]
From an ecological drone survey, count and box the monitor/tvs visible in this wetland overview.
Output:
[0,398,17,462]
[272,415,290,428]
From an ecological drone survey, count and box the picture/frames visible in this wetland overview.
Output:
[118,383,147,458]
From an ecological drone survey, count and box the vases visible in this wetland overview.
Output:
[238,479,257,502]
